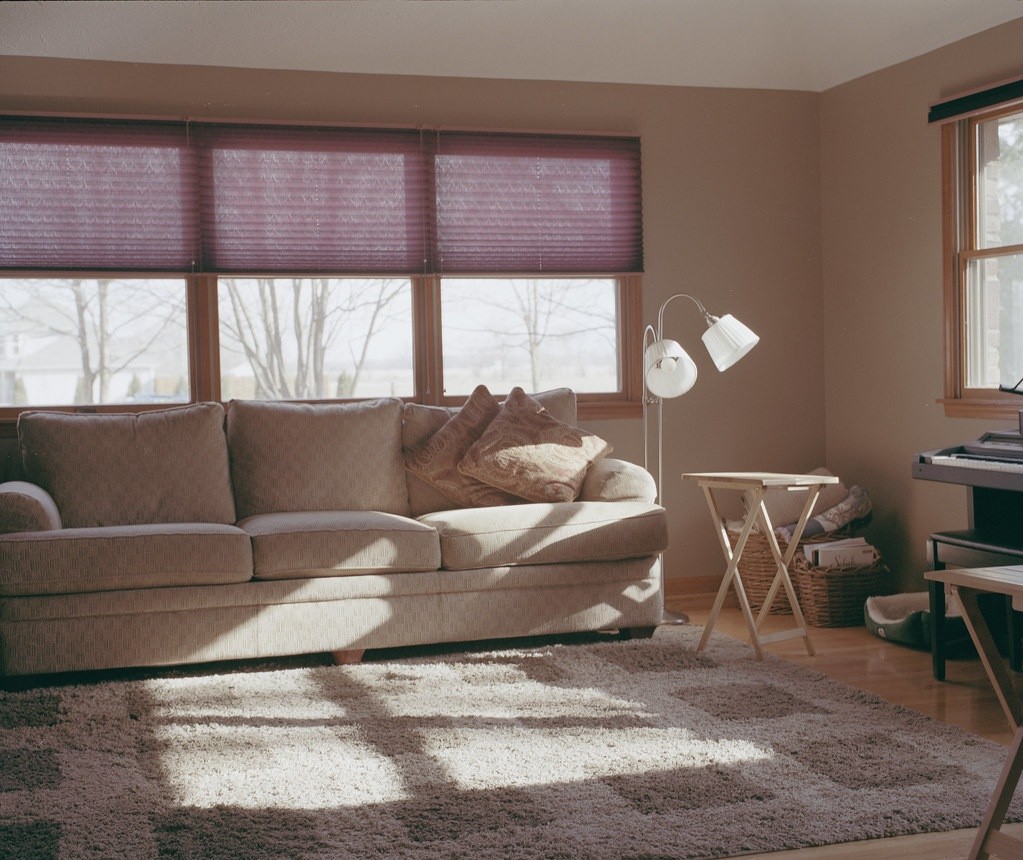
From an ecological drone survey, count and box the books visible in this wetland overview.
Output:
[803,537,875,569]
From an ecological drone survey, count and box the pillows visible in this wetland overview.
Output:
[401,384,526,508]
[16,401,235,527]
[403,389,577,515]
[457,386,614,503]
[226,400,410,520]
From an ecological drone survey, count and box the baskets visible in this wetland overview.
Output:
[792,551,884,628]
[725,529,847,616]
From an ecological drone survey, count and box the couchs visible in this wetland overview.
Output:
[0,388,669,679]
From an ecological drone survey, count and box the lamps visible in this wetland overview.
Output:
[642,294,761,625]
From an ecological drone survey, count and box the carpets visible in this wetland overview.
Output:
[0,625,1023,860]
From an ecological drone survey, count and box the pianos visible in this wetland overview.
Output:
[909,409,1023,648]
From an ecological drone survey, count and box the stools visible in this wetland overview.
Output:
[910,527,1023,683]
[924,564,1023,860]
[681,472,840,662]
